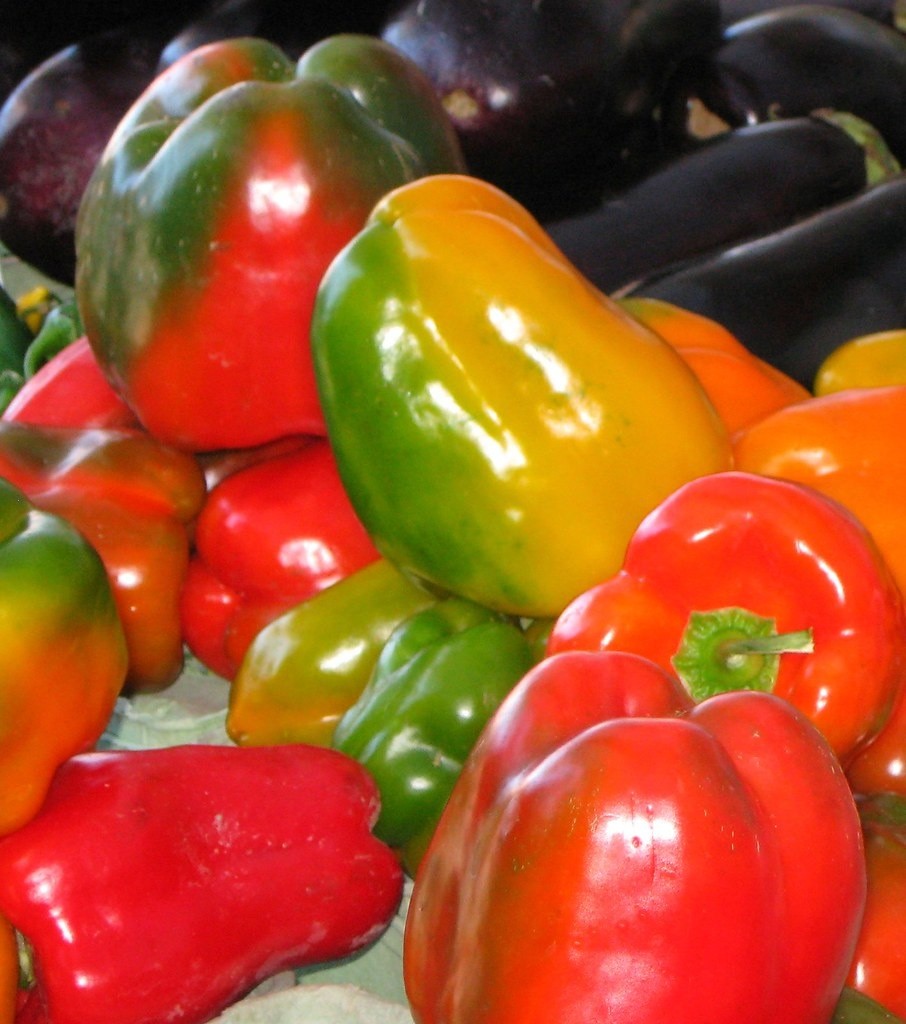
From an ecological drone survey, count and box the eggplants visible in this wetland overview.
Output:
[0,0,906,403]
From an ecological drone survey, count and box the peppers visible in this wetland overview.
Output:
[0,36,906,1024]
